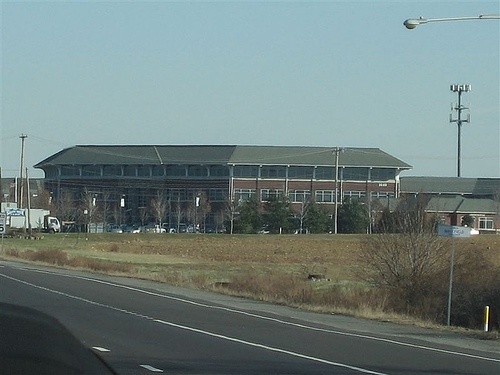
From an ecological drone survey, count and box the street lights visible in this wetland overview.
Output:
[403,14,499,30]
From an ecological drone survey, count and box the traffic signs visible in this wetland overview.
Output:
[436,223,480,238]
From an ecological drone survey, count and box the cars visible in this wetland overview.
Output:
[0,207,229,234]
